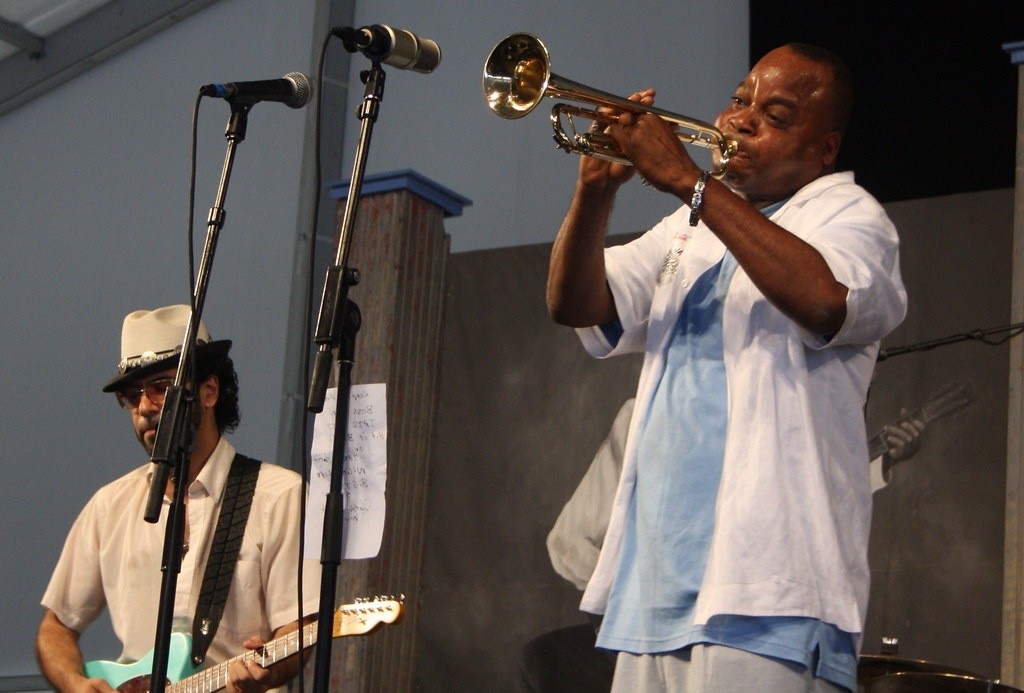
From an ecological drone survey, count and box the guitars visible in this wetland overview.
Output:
[82,588,407,693]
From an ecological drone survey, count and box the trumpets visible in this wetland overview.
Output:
[481,30,739,191]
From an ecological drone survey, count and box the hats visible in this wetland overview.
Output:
[102,304,233,393]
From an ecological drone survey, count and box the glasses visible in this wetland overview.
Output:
[115,377,175,411]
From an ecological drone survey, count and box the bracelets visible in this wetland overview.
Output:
[689,171,711,226]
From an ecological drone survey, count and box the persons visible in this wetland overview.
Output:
[519,395,927,693]
[35,304,318,693]
[547,40,904,693]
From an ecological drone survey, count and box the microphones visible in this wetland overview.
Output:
[202,71,314,109]
[332,23,442,74]
